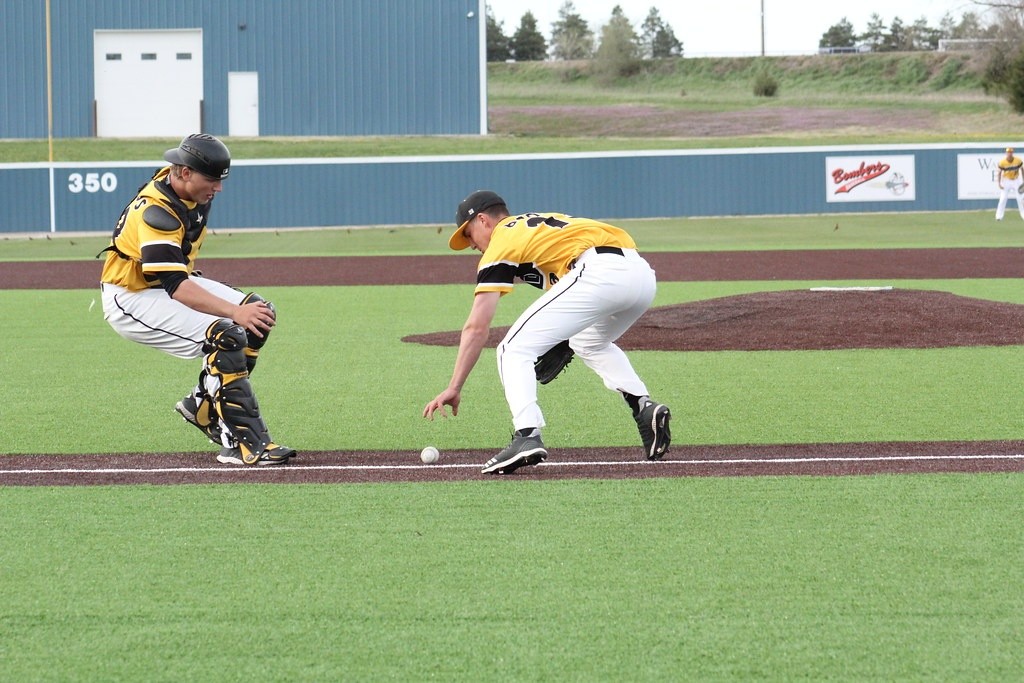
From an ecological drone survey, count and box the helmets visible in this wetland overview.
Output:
[1006,147,1013,152]
[163,133,231,179]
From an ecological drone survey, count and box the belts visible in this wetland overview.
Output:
[594,246,624,256]
[101,283,127,293]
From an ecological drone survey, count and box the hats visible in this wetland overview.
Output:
[448,189,506,251]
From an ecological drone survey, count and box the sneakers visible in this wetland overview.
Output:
[632,400,673,460]
[480,428,548,476]
[215,442,288,466]
[174,393,222,446]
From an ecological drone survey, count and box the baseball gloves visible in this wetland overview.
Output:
[1018,183,1024,194]
[534,340,574,384]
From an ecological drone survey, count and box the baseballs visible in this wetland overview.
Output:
[420,446,440,464]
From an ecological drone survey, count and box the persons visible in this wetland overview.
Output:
[99,134,297,464]
[996,148,1024,221]
[422,190,672,474]
